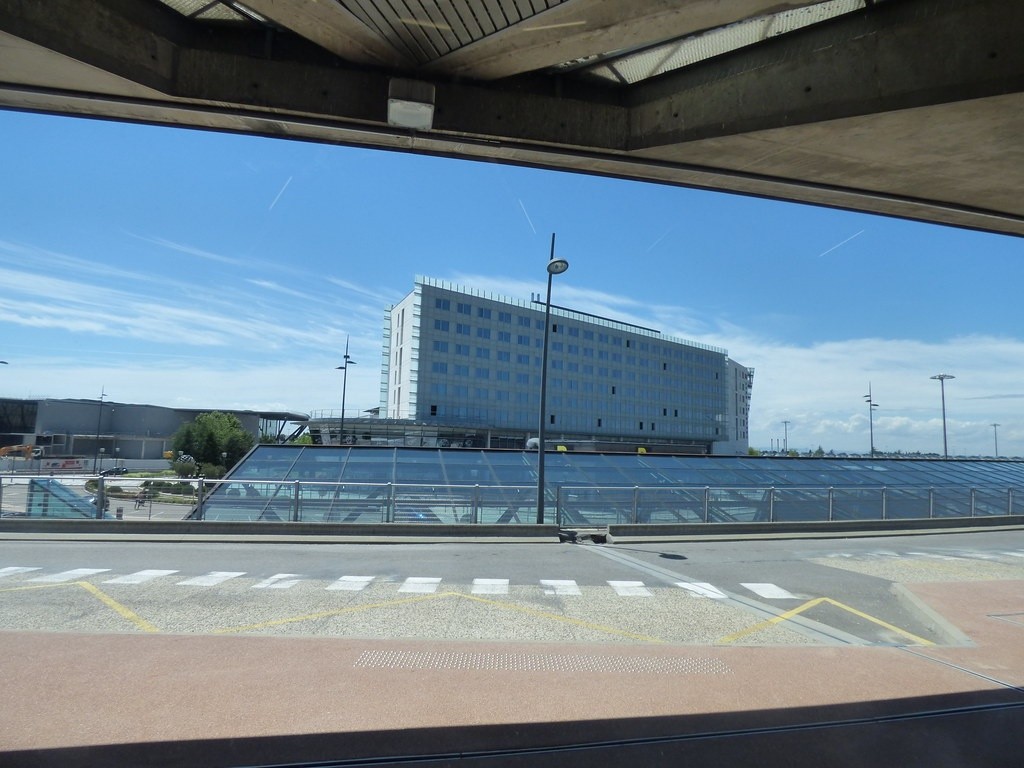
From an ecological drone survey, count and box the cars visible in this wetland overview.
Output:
[100,466,129,478]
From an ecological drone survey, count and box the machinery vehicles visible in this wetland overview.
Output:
[0,442,33,461]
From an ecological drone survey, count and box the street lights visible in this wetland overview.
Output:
[990,423,1003,458]
[862,380,879,457]
[537,232,569,524]
[335,333,356,444]
[932,372,957,459]
[221,452,227,480]
[782,420,791,456]
[92,384,107,476]
